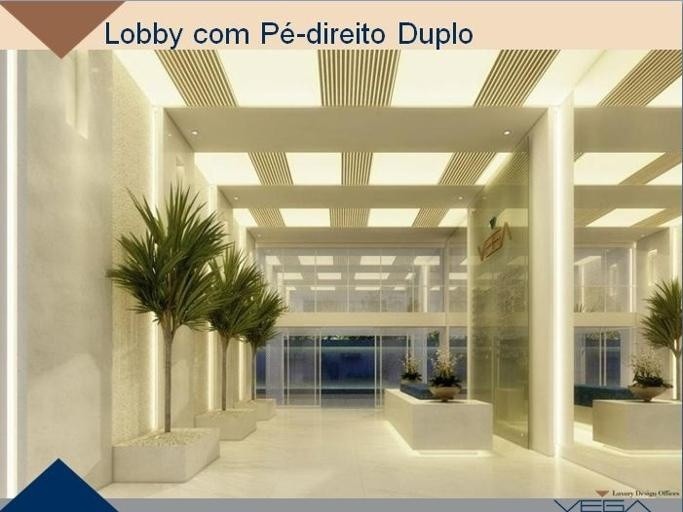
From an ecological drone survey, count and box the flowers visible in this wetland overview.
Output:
[400,354,422,383]
[427,345,463,390]
[625,347,673,389]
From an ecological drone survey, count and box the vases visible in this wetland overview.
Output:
[628,384,668,402]
[429,387,460,402]
